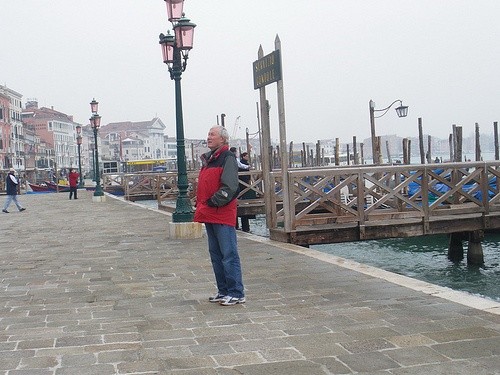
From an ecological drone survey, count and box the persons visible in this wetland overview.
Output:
[2,168,26,213]
[69,169,80,200]
[230,147,252,200]
[193,124,246,306]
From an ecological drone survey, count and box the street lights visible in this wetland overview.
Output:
[158,0,204,243]
[245,127,265,171]
[368,97,410,203]
[75,124,88,196]
[89,98,106,203]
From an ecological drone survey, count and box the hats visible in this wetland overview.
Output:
[9,168,15,171]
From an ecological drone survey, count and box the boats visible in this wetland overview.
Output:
[28,183,54,192]
[46,179,79,192]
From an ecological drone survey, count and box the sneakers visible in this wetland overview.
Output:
[209,293,225,302]
[219,295,246,306]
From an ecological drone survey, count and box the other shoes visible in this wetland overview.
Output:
[2,209,9,213]
[19,208,27,212]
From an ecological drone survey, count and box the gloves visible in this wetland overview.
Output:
[207,199,216,207]
